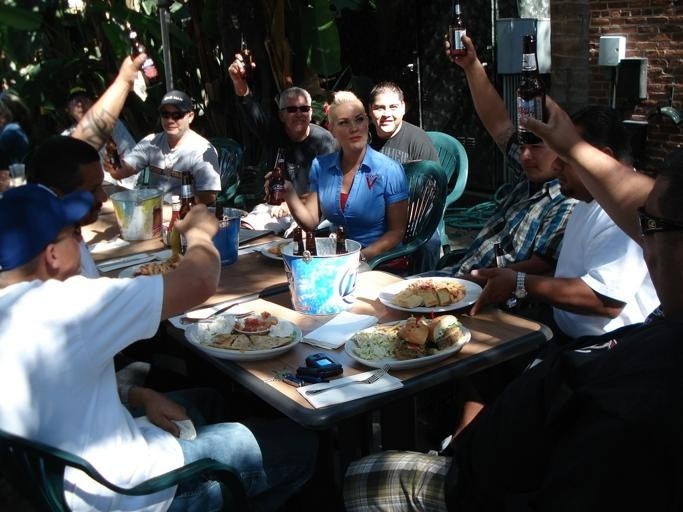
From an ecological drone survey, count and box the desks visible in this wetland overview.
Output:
[100,240,552,509]
[4,175,276,270]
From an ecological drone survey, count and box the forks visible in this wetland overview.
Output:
[305,364,392,395]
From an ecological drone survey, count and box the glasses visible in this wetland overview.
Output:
[69,98,93,108]
[278,104,312,116]
[633,204,683,239]
[158,109,189,120]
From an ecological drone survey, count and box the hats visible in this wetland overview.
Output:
[0,178,97,275]
[156,88,194,116]
[65,85,96,107]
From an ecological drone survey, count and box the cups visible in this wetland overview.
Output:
[6,164,25,184]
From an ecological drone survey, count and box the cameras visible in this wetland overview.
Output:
[305,352,342,380]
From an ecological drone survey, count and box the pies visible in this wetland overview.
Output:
[207,333,292,350]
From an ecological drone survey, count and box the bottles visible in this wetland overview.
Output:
[335,226,347,254]
[448,2,467,58]
[237,32,253,80]
[516,34,546,144]
[168,196,179,255]
[121,21,162,89]
[267,147,285,204]
[178,172,197,254]
[493,242,518,309]
[213,202,224,221]
[106,135,119,171]
[292,227,303,255]
[307,231,317,254]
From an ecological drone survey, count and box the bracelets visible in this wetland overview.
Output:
[360,252,366,262]
[120,384,136,409]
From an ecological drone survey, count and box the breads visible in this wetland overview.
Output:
[393,281,464,309]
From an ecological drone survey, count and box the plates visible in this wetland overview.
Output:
[261,239,289,260]
[118,260,178,277]
[345,321,471,370]
[379,276,482,313]
[183,315,301,360]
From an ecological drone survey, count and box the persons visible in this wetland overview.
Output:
[345,95,681,510]
[421,34,567,310]
[2,43,157,195]
[1,185,318,511]
[103,91,222,219]
[228,51,441,276]
[61,83,141,191]
[21,137,214,391]
[455,103,662,437]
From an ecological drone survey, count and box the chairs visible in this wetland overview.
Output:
[138,131,556,279]
[2,338,249,510]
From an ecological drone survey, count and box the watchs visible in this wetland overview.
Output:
[513,271,529,299]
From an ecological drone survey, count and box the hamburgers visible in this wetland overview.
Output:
[427,315,464,355]
[395,314,429,359]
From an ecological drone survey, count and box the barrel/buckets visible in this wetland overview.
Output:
[110,188,164,242]
[210,206,245,266]
[280,237,362,316]
